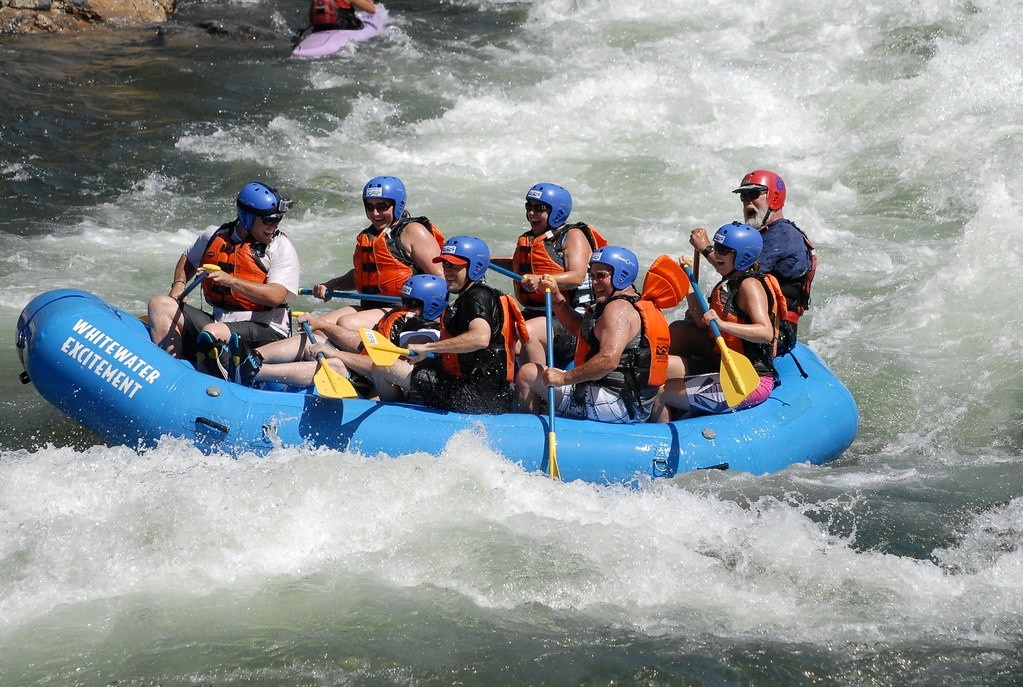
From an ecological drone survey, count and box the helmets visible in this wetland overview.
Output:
[441,235,490,280]
[236,182,282,230]
[712,222,763,270]
[525,183,572,227]
[363,175,406,218]
[401,274,449,320]
[741,169,785,210]
[589,245,638,288]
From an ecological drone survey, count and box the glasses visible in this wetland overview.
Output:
[740,188,768,200]
[364,201,394,212]
[525,202,551,212]
[713,243,735,256]
[401,296,423,308]
[258,214,281,225]
[588,270,613,280]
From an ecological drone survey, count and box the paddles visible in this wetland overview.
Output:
[298,286,401,305]
[693,250,700,284]
[684,258,762,408]
[542,274,562,482]
[291,310,360,400]
[489,261,524,282]
[357,327,441,368]
[139,263,222,324]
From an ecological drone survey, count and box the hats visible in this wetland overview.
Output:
[732,183,765,193]
[432,254,468,265]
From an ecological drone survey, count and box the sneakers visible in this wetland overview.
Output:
[228,332,263,386]
[197,331,233,381]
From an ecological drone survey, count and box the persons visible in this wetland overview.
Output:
[310,0,375,31]
[148,182,301,358]
[372,236,529,415]
[669,169,817,356]
[511,246,671,423]
[512,183,608,367]
[197,274,450,390]
[312,176,445,331]
[648,221,788,422]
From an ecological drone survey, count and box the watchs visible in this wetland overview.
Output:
[702,246,714,258]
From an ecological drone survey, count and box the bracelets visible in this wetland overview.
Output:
[166,278,186,289]
[552,299,564,305]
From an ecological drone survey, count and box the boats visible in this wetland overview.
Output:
[14,287,858,491]
[291,3,388,60]
